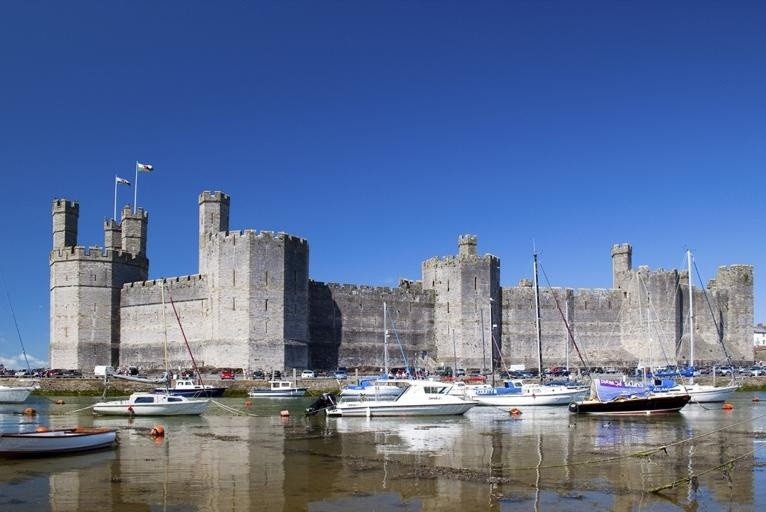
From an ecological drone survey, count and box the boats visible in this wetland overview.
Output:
[1,428,116,458]
[248,381,310,396]
[156,380,228,398]
[0,384,39,405]
[93,391,208,416]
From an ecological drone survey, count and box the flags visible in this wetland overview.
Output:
[137,162,153,174]
[118,177,132,187]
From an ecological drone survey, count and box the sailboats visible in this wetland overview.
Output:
[325,302,478,418]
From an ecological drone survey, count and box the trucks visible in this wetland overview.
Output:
[94,365,115,378]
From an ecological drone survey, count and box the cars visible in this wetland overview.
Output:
[301,369,315,380]
[15,368,82,378]
[254,371,281,381]
[742,365,766,376]
[221,371,235,380]
